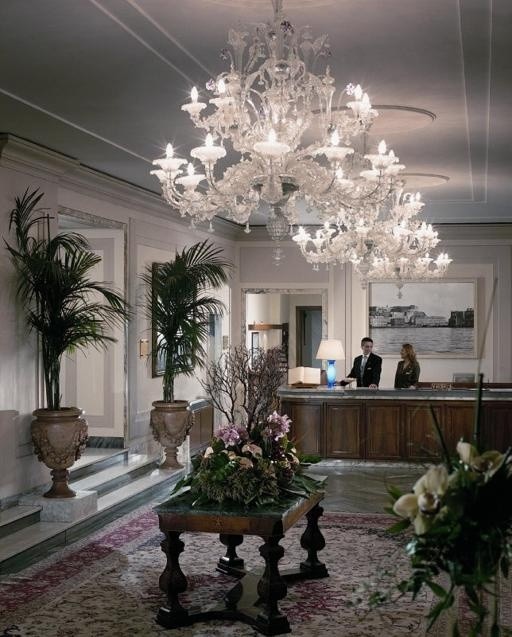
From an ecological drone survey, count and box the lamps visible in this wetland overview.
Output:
[315,339,345,388]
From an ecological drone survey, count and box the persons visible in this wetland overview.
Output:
[334,335,383,390]
[394,343,420,389]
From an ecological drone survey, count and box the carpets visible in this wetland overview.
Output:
[0,502,511,636]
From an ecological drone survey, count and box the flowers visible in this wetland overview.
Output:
[168,410,327,515]
[363,437,511,636]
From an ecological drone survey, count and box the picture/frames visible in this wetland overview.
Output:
[362,275,479,359]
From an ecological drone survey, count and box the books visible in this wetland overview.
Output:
[287,366,321,385]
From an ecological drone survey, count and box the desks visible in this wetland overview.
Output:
[152,472,321,636]
